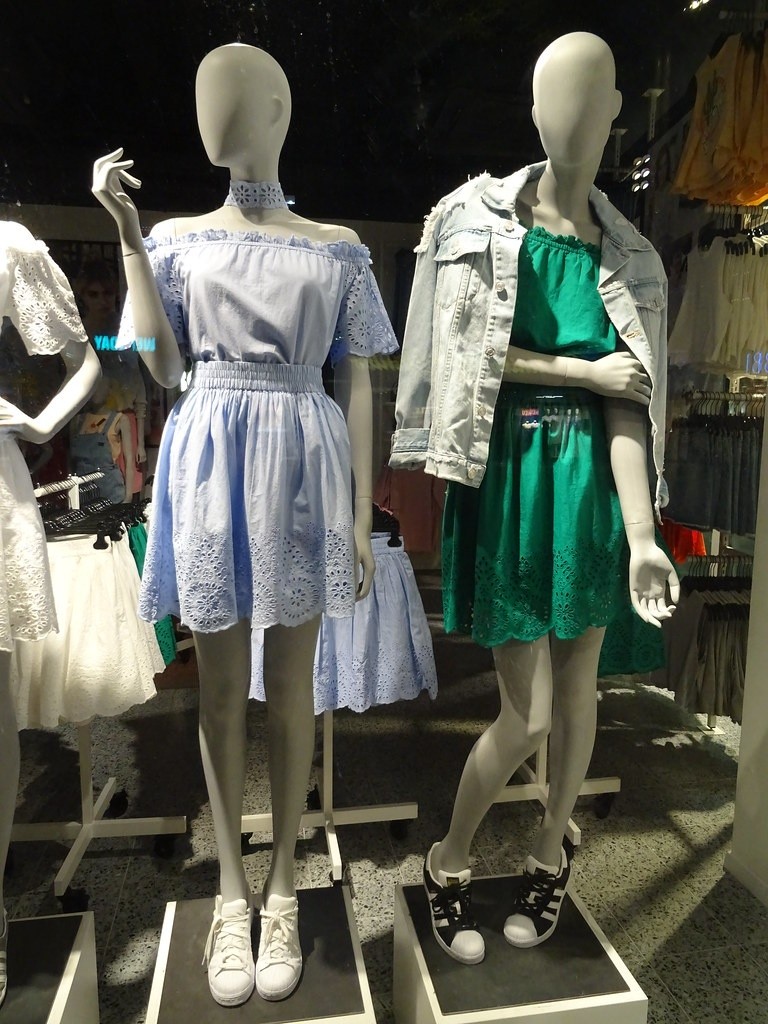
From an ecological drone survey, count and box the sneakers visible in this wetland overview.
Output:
[503,845,572,949]
[423,842,485,965]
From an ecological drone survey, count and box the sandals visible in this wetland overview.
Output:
[0,908,8,1007]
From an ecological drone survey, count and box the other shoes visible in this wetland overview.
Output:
[201,880,255,1007]
[256,878,302,1002]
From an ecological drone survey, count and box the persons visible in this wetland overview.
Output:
[419,26,683,970]
[0,219,108,1010]
[16,436,54,480]
[88,41,376,1010]
[92,350,148,506]
[53,247,123,340]
